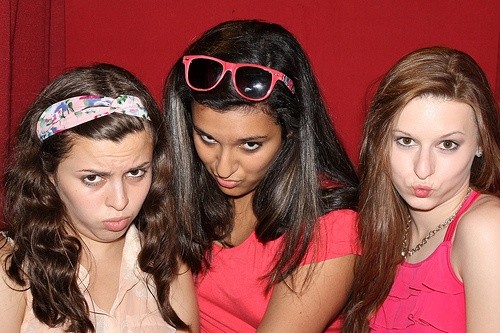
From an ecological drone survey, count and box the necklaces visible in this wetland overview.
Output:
[400,187,472,258]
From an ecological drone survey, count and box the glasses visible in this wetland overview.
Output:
[182,55,295,102]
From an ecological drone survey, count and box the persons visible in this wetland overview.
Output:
[1,61,198,332]
[161,18,364,333]
[326,45,500,333]
